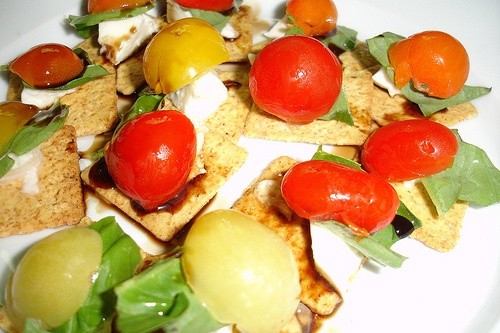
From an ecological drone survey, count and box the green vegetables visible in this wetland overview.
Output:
[1,0,499,333]
